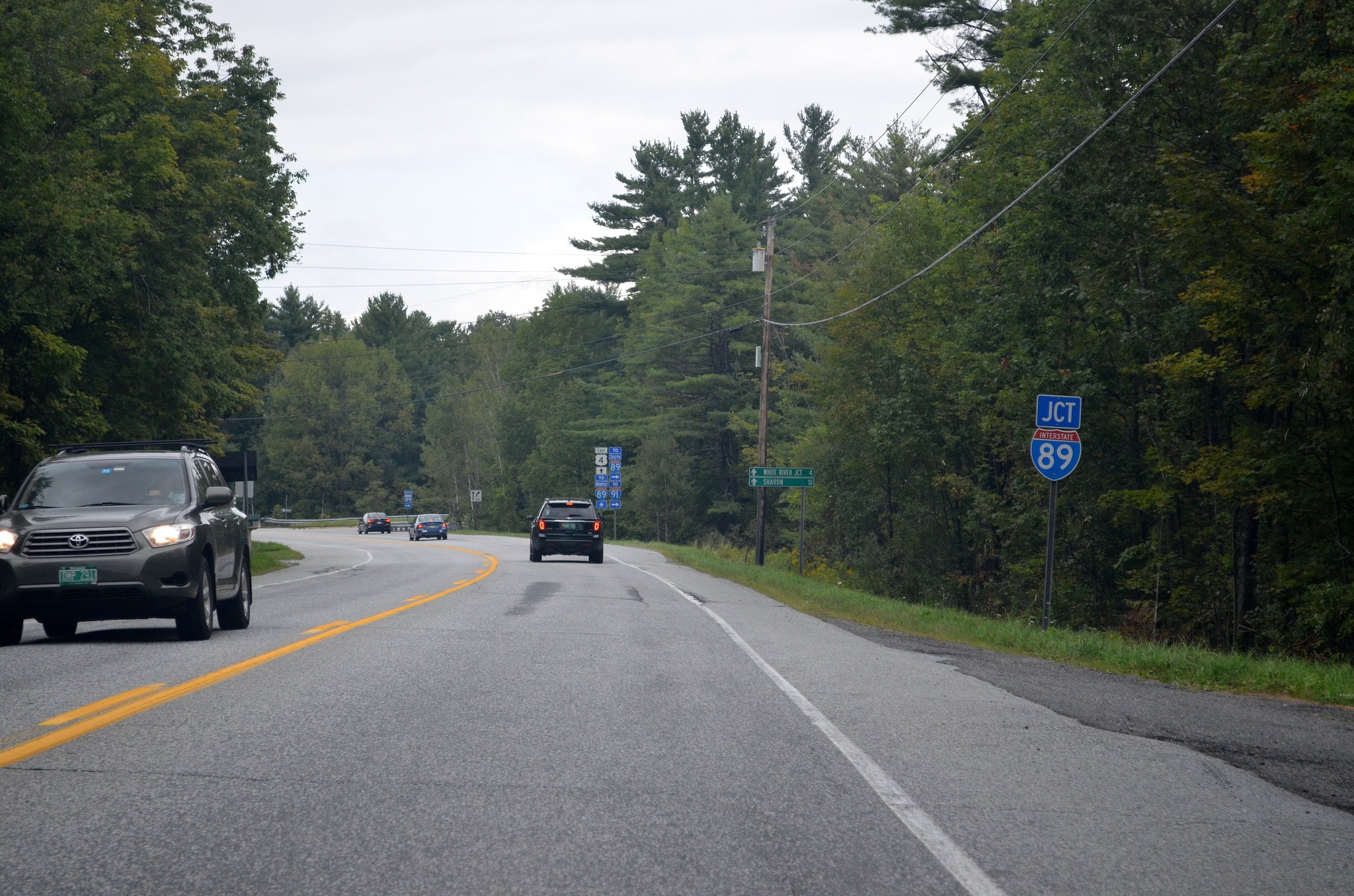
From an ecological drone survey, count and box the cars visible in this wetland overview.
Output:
[408,514,447,541]
[0,443,253,648]
[526,497,606,563]
[358,512,392,535]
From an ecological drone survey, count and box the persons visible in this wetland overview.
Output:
[146,464,185,505]
